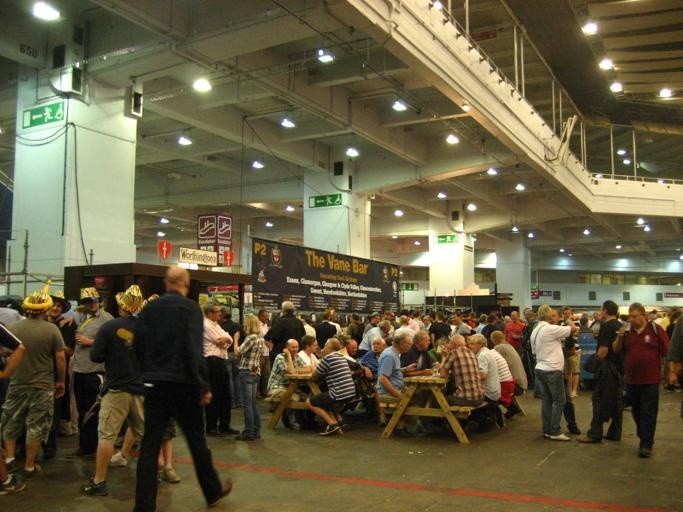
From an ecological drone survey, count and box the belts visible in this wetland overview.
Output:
[206,356,226,361]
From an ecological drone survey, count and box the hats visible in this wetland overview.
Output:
[134,293,160,316]
[370,311,379,317]
[77,286,103,307]
[114,284,143,313]
[20,279,52,314]
[49,290,71,314]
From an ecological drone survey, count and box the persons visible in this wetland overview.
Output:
[229,299,683,443]
[613,303,671,457]
[0,264,234,510]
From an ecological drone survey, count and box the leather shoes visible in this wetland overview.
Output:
[209,477,234,507]
[601,433,620,442]
[205,429,223,437]
[576,435,600,443]
[86,450,97,461]
[65,446,91,456]
[220,426,239,434]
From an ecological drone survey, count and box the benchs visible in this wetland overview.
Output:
[380,395,426,440]
[441,392,524,446]
[265,395,283,429]
[326,392,375,436]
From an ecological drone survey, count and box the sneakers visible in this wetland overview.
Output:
[503,408,521,419]
[70,424,78,433]
[567,425,579,433]
[253,430,260,438]
[544,433,549,438]
[663,383,674,391]
[0,474,26,496]
[79,478,107,496]
[639,446,651,458]
[4,456,19,473]
[319,424,339,435]
[108,452,126,466]
[550,433,570,440]
[235,431,254,441]
[675,384,681,392]
[22,463,45,480]
[58,421,72,435]
[337,420,346,429]
[163,468,180,483]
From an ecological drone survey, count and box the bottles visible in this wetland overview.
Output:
[622,319,632,337]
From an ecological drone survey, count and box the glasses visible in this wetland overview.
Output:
[210,308,221,314]
